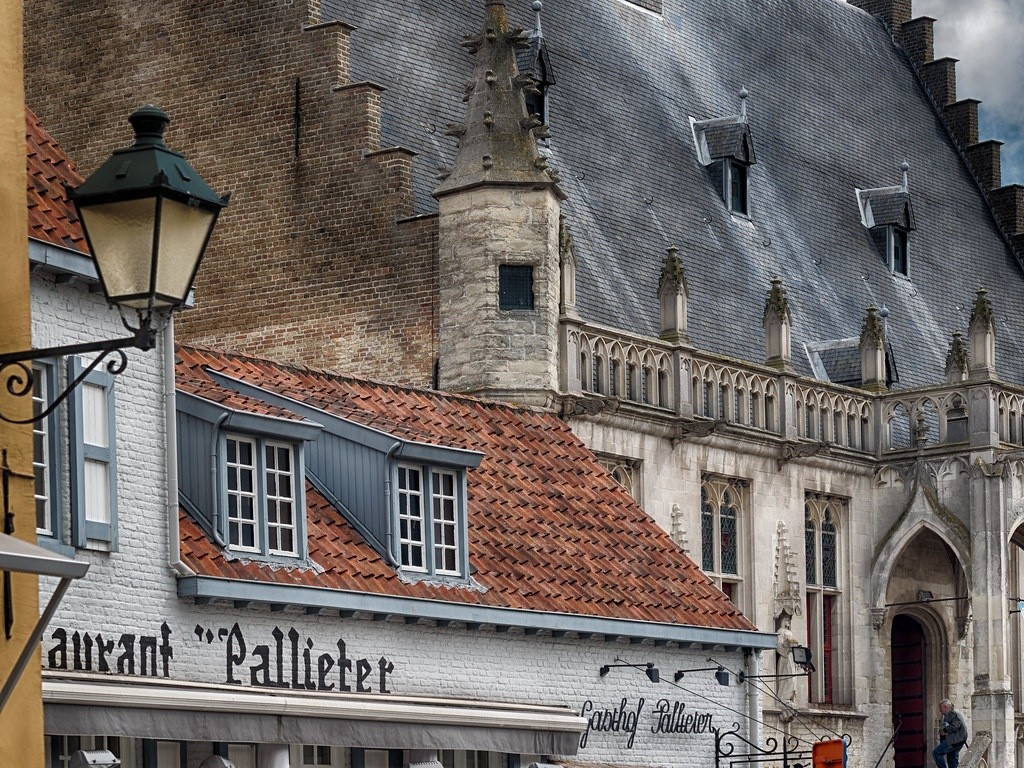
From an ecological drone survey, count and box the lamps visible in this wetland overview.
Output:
[674,666,729,686]
[68,748,121,768]
[739,647,815,685]
[600,661,660,683]
[1,108,231,427]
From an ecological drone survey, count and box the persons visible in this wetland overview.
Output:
[932,699,968,768]
[776,612,799,709]
